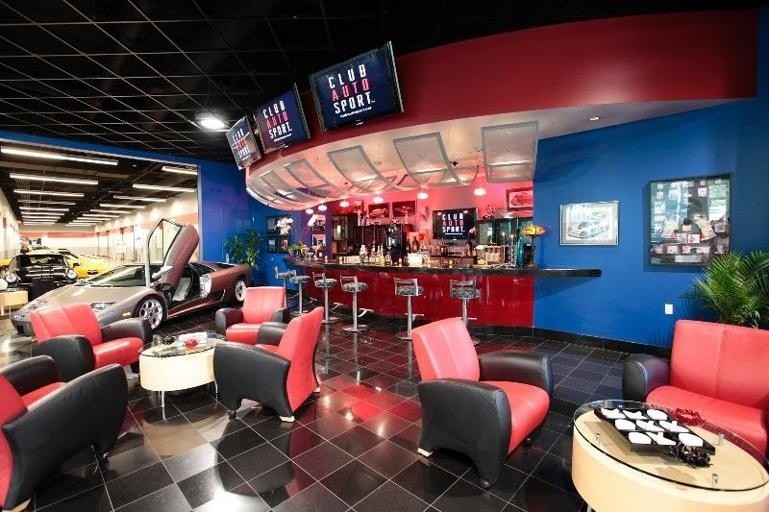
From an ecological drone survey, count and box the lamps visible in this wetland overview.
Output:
[305,190,314,215]
[416,177,429,200]
[373,193,384,205]
[318,194,328,212]
[473,172,487,196]
[1,147,198,228]
[340,199,349,208]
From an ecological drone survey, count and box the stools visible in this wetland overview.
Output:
[274,267,298,308]
[339,275,374,333]
[287,269,318,316]
[393,277,424,341]
[311,271,343,325]
[449,279,483,346]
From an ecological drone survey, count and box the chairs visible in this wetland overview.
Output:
[210,306,325,424]
[410,316,554,490]
[213,285,291,334]
[171,267,191,305]
[623,318,769,458]
[29,302,153,382]
[0,353,129,509]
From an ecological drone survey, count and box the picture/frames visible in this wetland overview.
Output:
[266,215,290,254]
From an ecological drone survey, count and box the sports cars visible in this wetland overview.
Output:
[9,217,253,339]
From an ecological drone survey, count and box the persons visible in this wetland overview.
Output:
[20,243,29,253]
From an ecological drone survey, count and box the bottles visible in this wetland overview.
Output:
[440,241,448,255]
[413,240,418,252]
[512,230,523,268]
[325,256,328,262]
[405,241,410,250]
[411,236,417,250]
[360,244,392,266]
[465,232,472,255]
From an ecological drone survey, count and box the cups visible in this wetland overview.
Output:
[153,334,160,344]
[337,255,359,262]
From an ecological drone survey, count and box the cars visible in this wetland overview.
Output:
[1,255,80,300]
[1,248,112,280]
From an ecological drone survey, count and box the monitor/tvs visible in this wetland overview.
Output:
[225,115,264,172]
[252,81,312,155]
[432,208,478,243]
[307,41,405,133]
